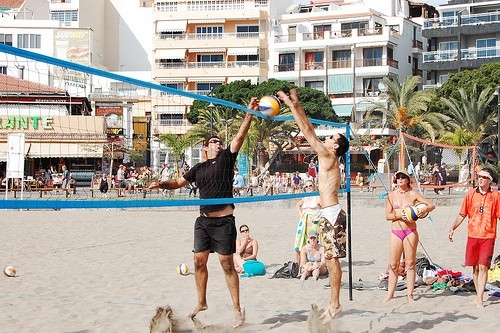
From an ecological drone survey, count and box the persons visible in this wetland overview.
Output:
[448,167,500,310]
[0,155,448,196]
[384,170,435,303]
[277,88,350,325]
[149,97,259,328]
[233,224,329,283]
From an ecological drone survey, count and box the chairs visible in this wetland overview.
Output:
[434,55,442,60]
[450,53,458,59]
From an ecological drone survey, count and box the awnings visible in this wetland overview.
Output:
[0,143,105,162]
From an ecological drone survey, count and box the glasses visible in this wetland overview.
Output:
[208,140,223,145]
[330,135,338,144]
[240,229,248,233]
[304,184,312,189]
[478,175,489,179]
[396,174,407,179]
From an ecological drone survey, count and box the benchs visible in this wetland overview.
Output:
[134,187,159,198]
[350,184,370,191]
[0,188,25,198]
[88,188,126,197]
[31,188,75,198]
[371,185,384,192]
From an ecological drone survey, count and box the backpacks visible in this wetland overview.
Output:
[268,261,300,280]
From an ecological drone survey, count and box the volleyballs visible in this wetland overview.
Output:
[177,263,189,275]
[415,202,429,219]
[403,206,419,222]
[258,94,282,116]
[4,265,16,277]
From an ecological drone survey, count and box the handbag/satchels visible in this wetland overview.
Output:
[240,258,267,278]
[414,252,431,276]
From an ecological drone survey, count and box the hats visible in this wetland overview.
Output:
[394,170,410,179]
[309,230,318,239]
